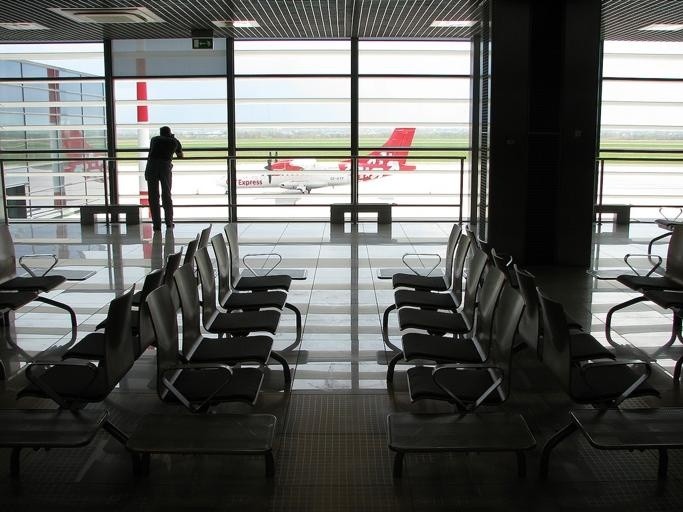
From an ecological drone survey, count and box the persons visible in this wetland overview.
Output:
[150,230,174,271]
[144,126,182,230]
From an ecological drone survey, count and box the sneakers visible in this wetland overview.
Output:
[167,224,174,228]
[153,226,161,231]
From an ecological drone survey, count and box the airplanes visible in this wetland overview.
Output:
[217,128,417,195]
[59,117,108,176]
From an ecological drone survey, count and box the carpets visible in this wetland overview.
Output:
[240,267,309,281]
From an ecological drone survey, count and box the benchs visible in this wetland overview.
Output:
[77,202,140,225]
[329,222,396,245]
[593,202,633,225]
[329,201,393,226]
